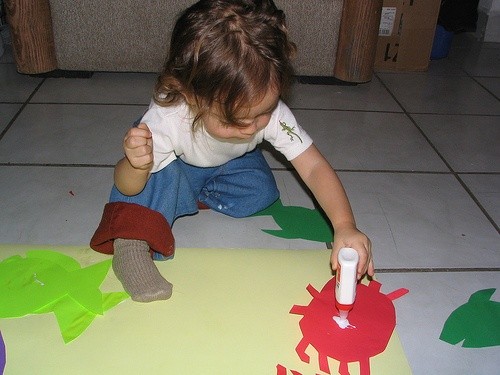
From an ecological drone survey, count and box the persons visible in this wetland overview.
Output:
[91,0,374,302]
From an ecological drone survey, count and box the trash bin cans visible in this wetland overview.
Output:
[430,0,480,60]
